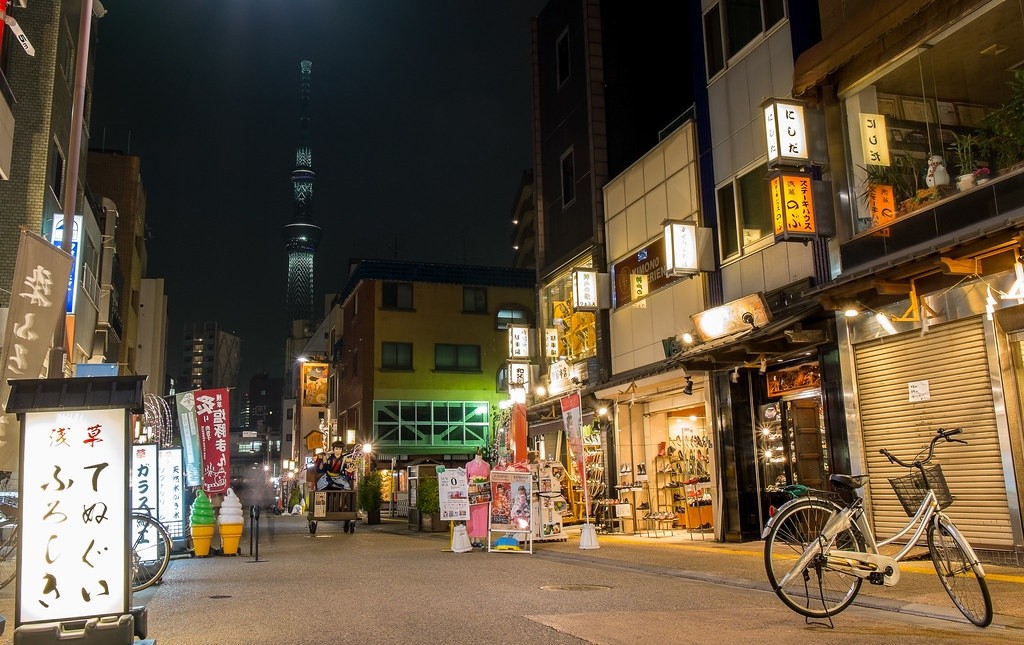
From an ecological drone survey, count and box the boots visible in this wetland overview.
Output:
[658,442,666,456]
[668,446,676,455]
[621,463,632,473]
[637,464,646,475]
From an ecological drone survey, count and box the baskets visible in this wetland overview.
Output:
[887,464,953,517]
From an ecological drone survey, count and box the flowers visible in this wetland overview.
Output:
[975,167,993,182]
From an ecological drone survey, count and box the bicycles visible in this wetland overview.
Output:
[761,427,994,628]
[0,502,174,593]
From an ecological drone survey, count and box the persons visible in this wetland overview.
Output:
[492,460,515,471]
[492,483,529,524]
[465,452,490,547]
[316,496,325,505]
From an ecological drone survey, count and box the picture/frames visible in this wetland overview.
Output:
[978,179,988,186]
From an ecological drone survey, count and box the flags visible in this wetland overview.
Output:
[0,225,75,472]
[193,388,230,497]
[176,389,202,486]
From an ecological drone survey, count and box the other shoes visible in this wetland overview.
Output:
[660,464,672,473]
[633,481,642,488]
[614,481,625,489]
[637,502,649,510]
[623,482,632,488]
[682,477,699,485]
[600,498,619,505]
[687,489,703,498]
[694,522,714,530]
[642,511,675,520]
[663,481,677,489]
[698,475,710,482]
[673,493,685,501]
[620,498,629,504]
[689,499,712,507]
[670,435,712,475]
[675,506,684,513]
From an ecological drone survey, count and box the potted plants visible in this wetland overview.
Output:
[415,474,451,532]
[855,74,1023,228]
[355,473,383,525]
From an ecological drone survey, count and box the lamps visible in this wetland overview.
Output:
[742,312,759,334]
[684,377,693,395]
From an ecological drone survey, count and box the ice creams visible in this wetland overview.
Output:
[189,489,216,556]
[218,488,244,554]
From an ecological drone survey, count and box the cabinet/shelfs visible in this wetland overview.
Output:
[613,437,715,542]
